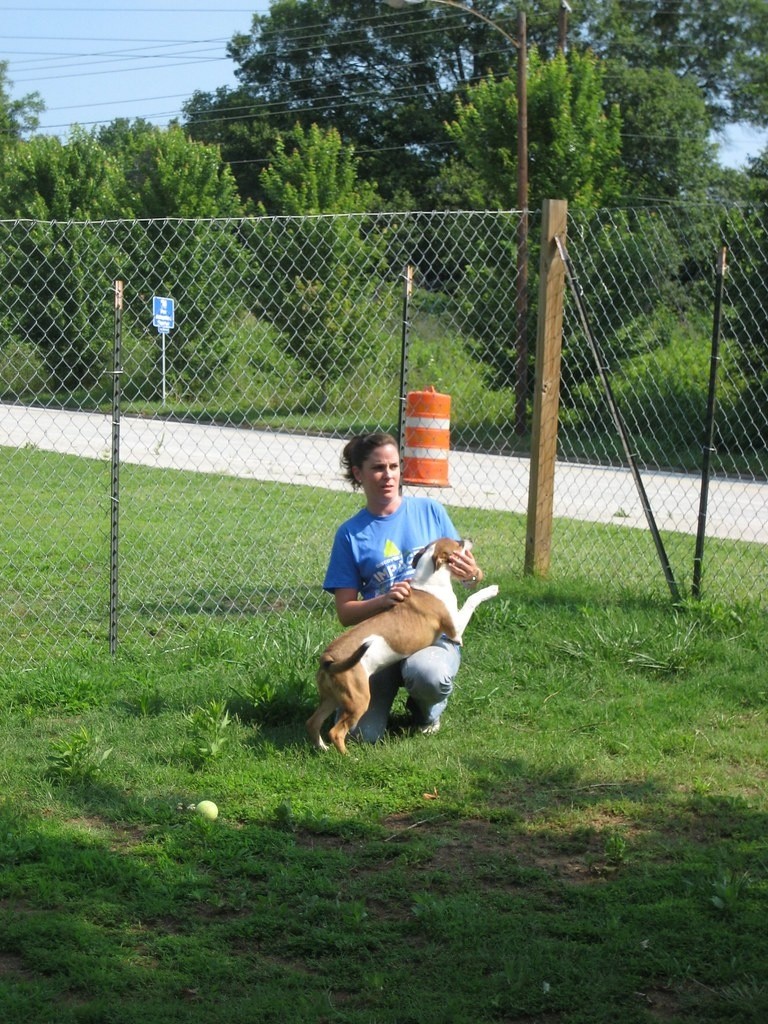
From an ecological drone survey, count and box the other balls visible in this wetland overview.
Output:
[195,800,219,821]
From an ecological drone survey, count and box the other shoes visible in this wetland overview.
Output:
[418,716,439,735]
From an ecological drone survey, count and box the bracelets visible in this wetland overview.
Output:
[463,570,479,586]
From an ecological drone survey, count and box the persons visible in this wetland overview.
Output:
[324,433,484,742]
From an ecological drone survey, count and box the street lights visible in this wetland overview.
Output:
[384,0,528,438]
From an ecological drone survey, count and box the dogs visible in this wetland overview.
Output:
[304,537,499,760]
